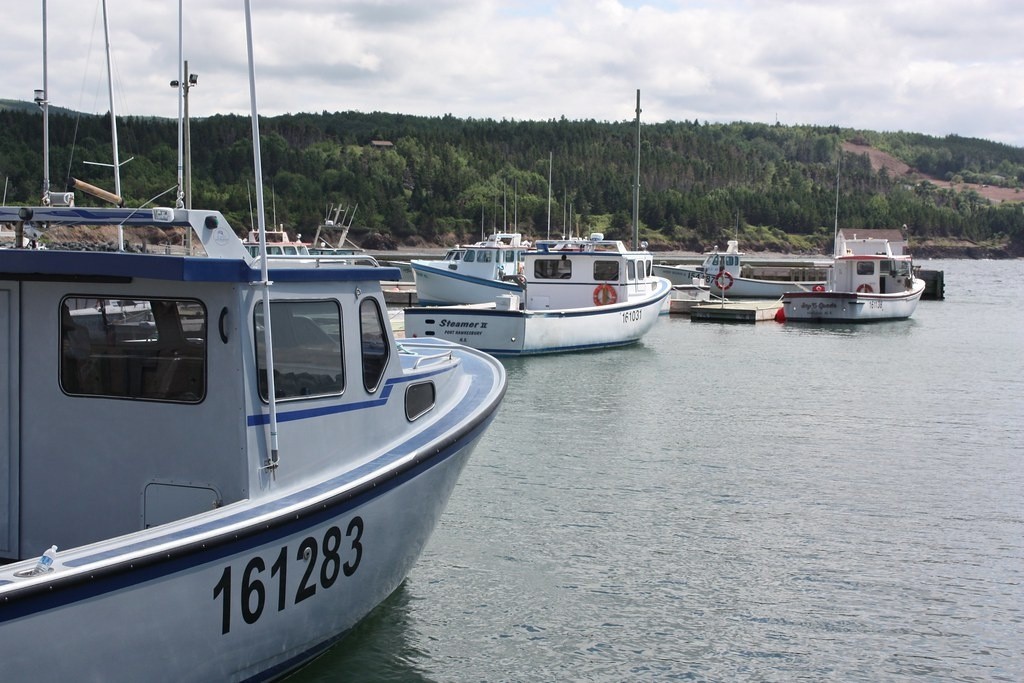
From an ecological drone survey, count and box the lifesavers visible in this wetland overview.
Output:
[715,272,733,290]
[593,284,616,306]
[856,284,873,293]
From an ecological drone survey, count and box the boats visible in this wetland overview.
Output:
[651,208,828,299]
[404,234,673,356]
[410,152,628,306]
[0,0,417,330]
[781,161,926,324]
[0,0,511,683]
